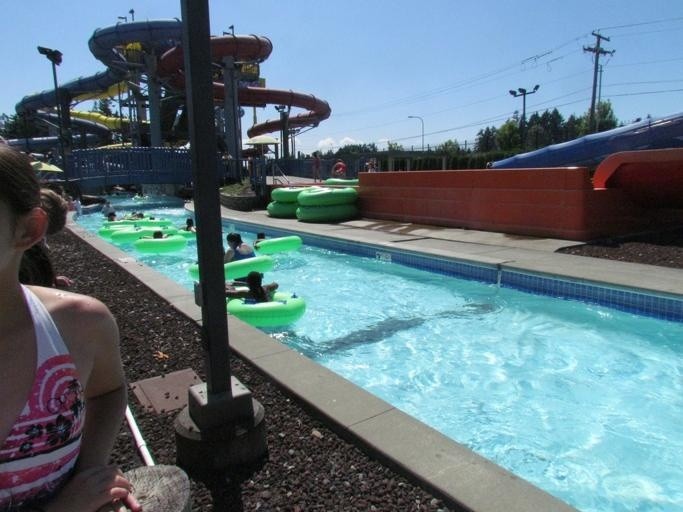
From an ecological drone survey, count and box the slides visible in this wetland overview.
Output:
[487,112,683,169]
[0,17,330,149]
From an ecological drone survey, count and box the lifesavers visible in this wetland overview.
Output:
[226,290,306,326]
[189,255,272,280]
[326,161,361,185]
[98,213,196,250]
[267,186,358,224]
[254,235,302,252]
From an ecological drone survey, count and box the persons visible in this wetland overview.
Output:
[101,199,118,221]
[18,188,74,291]
[152,230,163,240]
[1,145,142,511]
[225,232,256,262]
[179,218,196,233]
[131,211,143,219]
[256,232,266,242]
[224,272,278,303]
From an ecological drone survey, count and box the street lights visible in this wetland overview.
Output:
[36,45,71,182]
[509,84,540,151]
[408,115,424,153]
